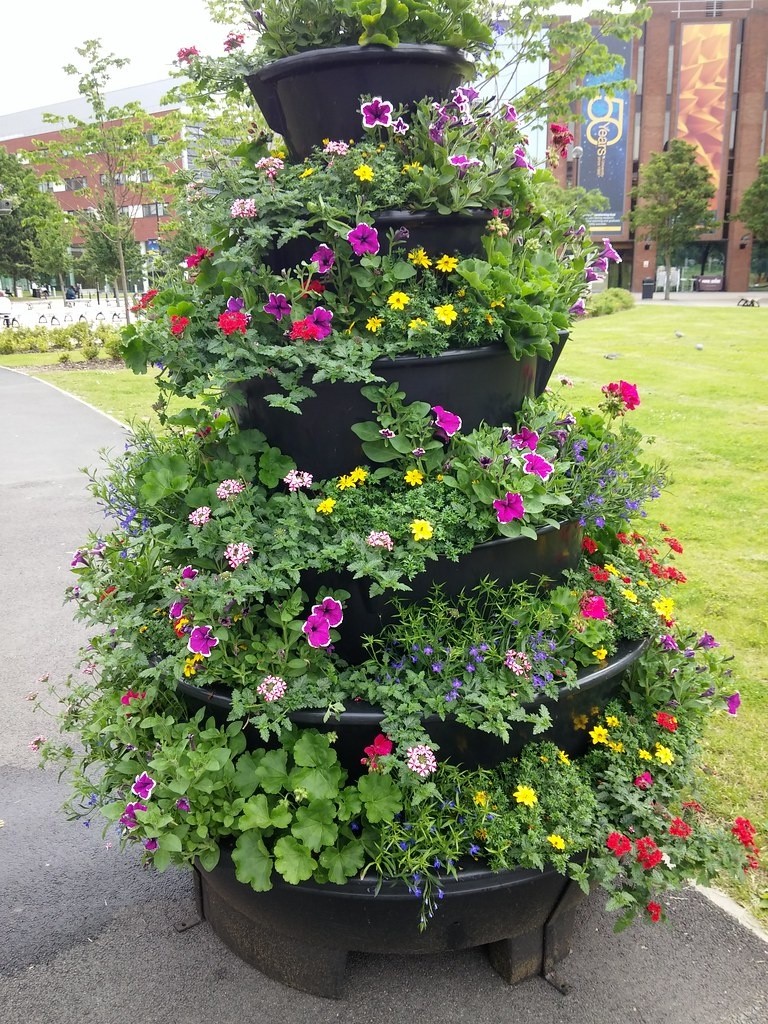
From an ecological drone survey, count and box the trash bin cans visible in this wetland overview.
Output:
[34,288,41,298]
[641,278,655,299]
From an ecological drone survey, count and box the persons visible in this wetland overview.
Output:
[4,286,13,298]
[32,281,50,298]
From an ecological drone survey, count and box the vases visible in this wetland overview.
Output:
[148,44,653,1001]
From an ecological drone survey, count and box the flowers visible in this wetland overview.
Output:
[38,0,766,978]
